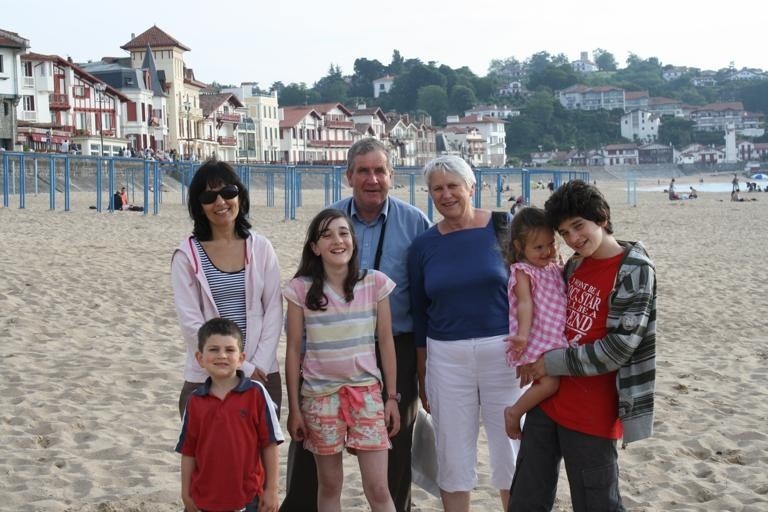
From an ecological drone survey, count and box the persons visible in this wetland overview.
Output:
[0,129,218,165]
[119,187,129,206]
[284,136,437,510]
[502,207,569,440]
[409,152,521,510]
[286,207,404,511]
[390,169,768,211]
[107,191,123,210]
[174,316,286,510]
[168,161,285,437]
[503,175,661,512]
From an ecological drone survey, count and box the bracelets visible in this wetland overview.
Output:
[383,390,403,403]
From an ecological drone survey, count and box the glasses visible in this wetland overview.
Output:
[199,184,239,204]
